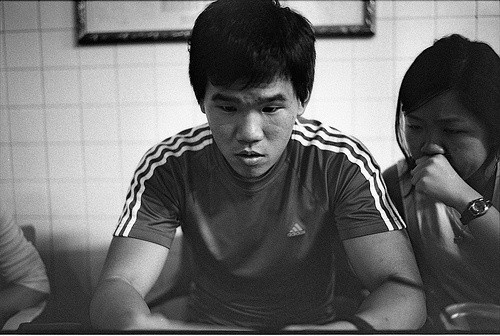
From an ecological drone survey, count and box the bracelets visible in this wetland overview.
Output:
[320,314,376,331]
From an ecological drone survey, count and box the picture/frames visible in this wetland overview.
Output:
[73,0,377,47]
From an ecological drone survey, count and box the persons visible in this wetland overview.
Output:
[382,34,500,332]
[89,0,427,331]
[0,207,51,330]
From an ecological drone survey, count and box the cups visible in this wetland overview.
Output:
[438,302,500,331]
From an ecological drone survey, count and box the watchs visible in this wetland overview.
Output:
[459,197,493,226]
